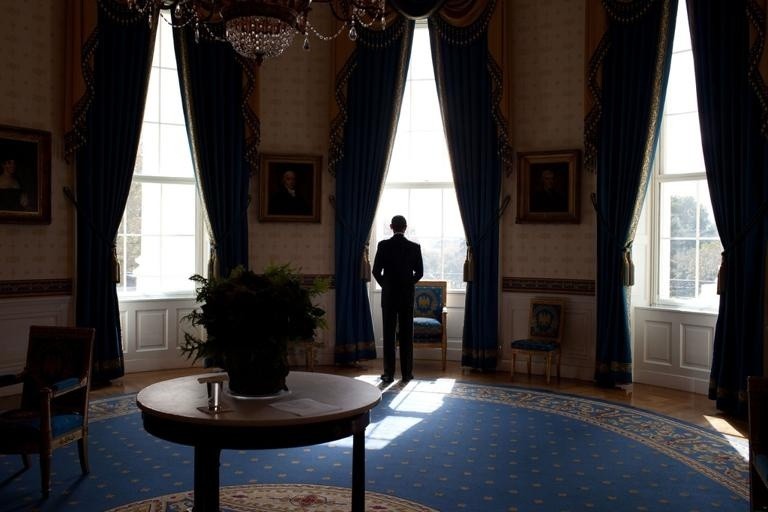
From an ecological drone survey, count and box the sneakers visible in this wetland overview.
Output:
[381,375,414,383]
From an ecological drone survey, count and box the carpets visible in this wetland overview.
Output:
[0,379,753,512]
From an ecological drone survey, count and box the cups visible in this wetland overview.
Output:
[206,380,223,412]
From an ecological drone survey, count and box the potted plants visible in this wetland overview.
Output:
[178,255,334,396]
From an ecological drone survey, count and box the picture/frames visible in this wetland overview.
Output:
[258,151,323,224]
[1,123,53,226]
[514,148,582,225]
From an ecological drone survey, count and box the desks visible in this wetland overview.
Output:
[136,369,382,511]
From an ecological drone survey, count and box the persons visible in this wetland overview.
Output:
[0,155,34,213]
[530,168,565,214]
[271,169,311,215]
[371,214,424,381]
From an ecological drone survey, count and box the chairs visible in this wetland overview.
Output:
[0,325,100,497]
[509,298,567,387]
[395,281,448,371]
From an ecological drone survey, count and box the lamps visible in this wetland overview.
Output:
[130,0,387,66]
